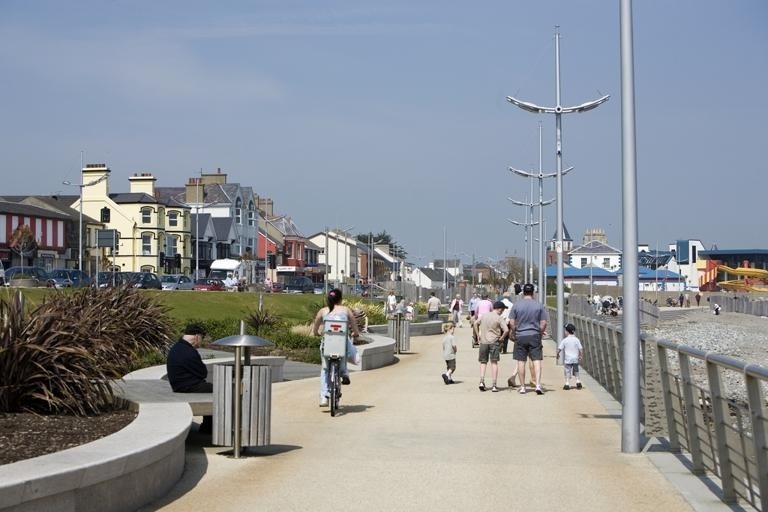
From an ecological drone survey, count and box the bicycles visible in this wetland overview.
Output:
[314,330,359,416]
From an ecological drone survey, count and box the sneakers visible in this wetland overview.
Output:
[562,384,570,390]
[449,379,455,384]
[442,373,449,385]
[341,372,351,385]
[535,385,544,395]
[491,387,499,392]
[519,386,527,393]
[319,397,329,407]
[478,383,488,391]
[577,382,582,388]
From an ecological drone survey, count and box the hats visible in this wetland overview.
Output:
[184,324,207,335]
[493,300,508,309]
[563,323,576,334]
[523,284,534,291]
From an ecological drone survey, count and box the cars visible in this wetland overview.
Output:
[0,266,53,288]
[194,276,225,291]
[109,272,163,292]
[48,268,91,288]
[158,273,195,291]
[89,271,123,288]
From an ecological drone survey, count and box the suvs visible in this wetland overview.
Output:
[283,276,314,294]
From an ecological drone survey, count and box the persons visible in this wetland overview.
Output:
[386,292,415,321]
[450,294,464,327]
[427,293,441,321]
[584,290,623,319]
[557,324,584,390]
[711,303,725,316]
[667,291,687,309]
[468,294,511,391]
[314,290,358,409]
[695,292,702,307]
[167,324,213,437]
[225,273,248,293]
[442,323,457,385]
[509,284,547,394]
[259,278,281,293]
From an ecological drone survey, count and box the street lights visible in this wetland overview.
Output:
[249,200,287,280]
[324,222,355,288]
[506,197,558,288]
[505,191,547,288]
[670,245,687,297]
[515,166,546,289]
[645,240,665,292]
[507,217,541,286]
[508,120,575,305]
[580,230,603,305]
[506,119,575,336]
[507,168,555,312]
[245,193,286,278]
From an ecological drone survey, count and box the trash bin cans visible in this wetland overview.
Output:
[210,334,274,449]
[388,308,412,353]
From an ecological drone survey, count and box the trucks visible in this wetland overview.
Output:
[208,258,243,286]
